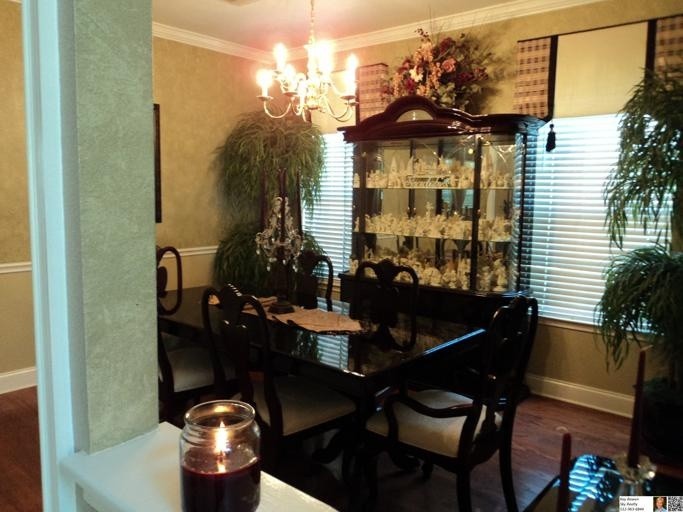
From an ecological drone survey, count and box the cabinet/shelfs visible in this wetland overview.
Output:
[335,93,543,335]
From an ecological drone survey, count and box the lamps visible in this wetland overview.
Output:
[254,0,361,125]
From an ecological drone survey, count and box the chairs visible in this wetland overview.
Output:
[155,243,539,512]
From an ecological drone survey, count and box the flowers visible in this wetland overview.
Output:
[379,8,509,111]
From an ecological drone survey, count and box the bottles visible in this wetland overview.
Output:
[179,399,262,511]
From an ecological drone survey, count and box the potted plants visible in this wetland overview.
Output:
[592,56,682,469]
